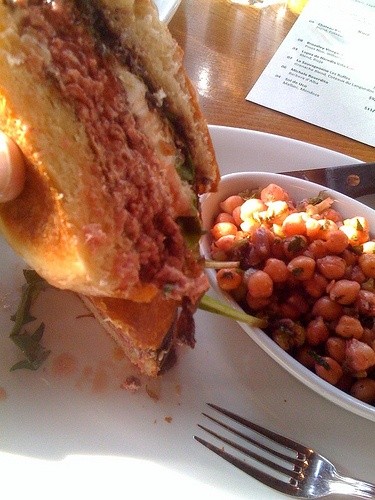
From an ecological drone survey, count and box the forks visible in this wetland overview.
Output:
[194,404,375,500]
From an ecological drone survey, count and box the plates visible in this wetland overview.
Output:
[1,125,375,500]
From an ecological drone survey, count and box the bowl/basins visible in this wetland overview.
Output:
[199,172,375,420]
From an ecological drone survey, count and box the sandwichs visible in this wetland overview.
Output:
[74,292,200,378]
[0,0,222,304]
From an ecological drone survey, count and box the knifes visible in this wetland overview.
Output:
[273,164,375,200]
